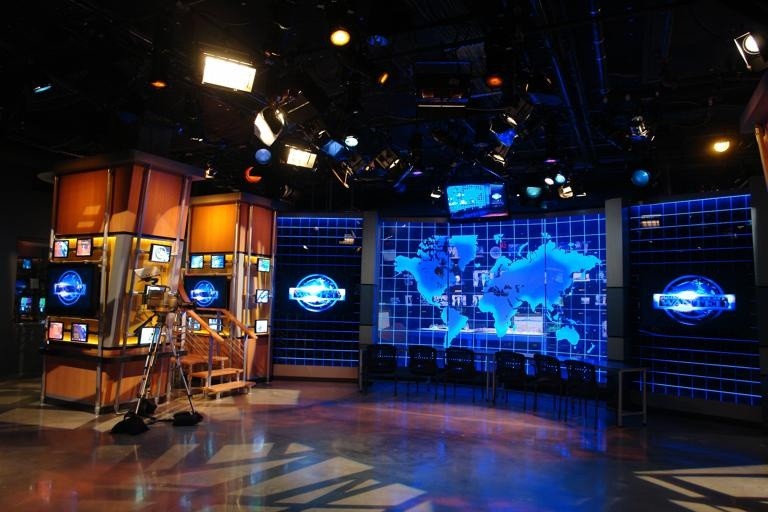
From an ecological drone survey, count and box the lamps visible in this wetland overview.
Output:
[183,25,768,199]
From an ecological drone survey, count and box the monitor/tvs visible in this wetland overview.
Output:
[211,254,225,269]
[142,284,166,304]
[255,288,269,305]
[254,319,268,333]
[52,240,69,258]
[47,321,64,341]
[149,243,172,263]
[75,237,93,257]
[442,179,511,220]
[182,274,229,315]
[189,254,204,269]
[138,327,162,346]
[70,322,88,342]
[256,258,270,273]
[44,260,99,318]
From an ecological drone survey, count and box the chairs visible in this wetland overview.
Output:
[364,344,643,428]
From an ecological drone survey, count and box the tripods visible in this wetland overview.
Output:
[135,313,196,418]
